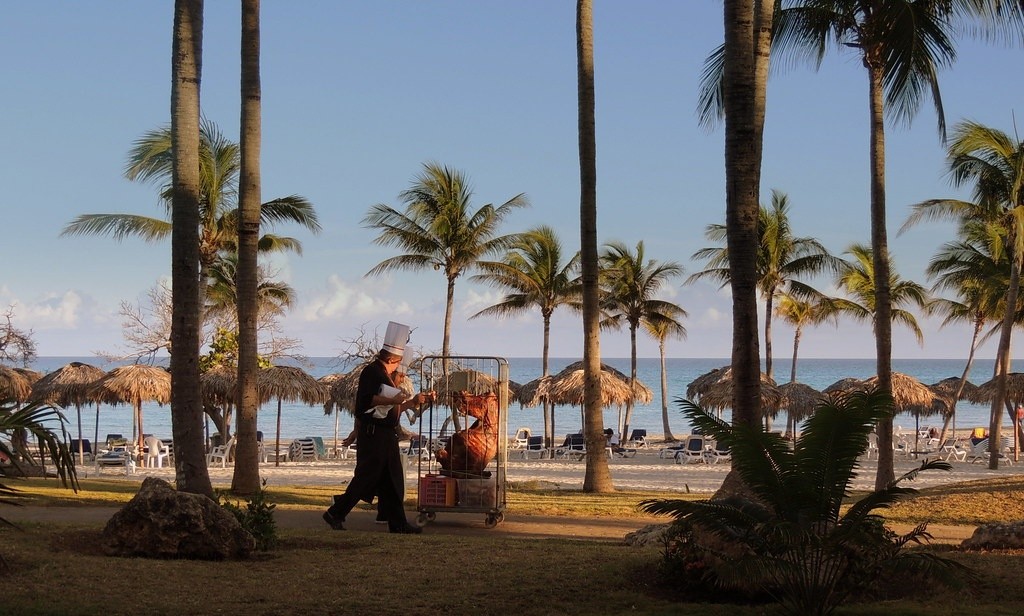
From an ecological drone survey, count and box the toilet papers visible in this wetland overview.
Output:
[364,384,401,419]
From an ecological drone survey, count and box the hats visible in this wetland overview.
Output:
[396,346,413,373]
[382,321,410,356]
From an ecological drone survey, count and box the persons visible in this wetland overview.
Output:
[929,427,940,439]
[604,428,620,452]
[1017,405,1024,427]
[341,347,436,524]
[322,321,422,534]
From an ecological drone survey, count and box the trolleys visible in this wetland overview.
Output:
[413,354,508,530]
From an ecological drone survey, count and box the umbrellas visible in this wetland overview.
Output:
[198,365,332,467]
[931,377,980,438]
[969,372,1024,461]
[327,362,523,429]
[0,362,171,468]
[684,364,826,452]
[517,360,655,457]
[821,371,951,459]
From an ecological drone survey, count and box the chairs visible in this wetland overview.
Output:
[510,427,648,462]
[205,435,236,469]
[257,431,358,463]
[400,434,430,465]
[67,434,176,482]
[658,429,732,466]
[865,424,1016,469]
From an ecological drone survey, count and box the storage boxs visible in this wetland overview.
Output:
[419,467,502,509]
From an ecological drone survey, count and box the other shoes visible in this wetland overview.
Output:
[322,512,346,530]
[334,495,346,522]
[389,522,422,534]
[376,514,389,521]
